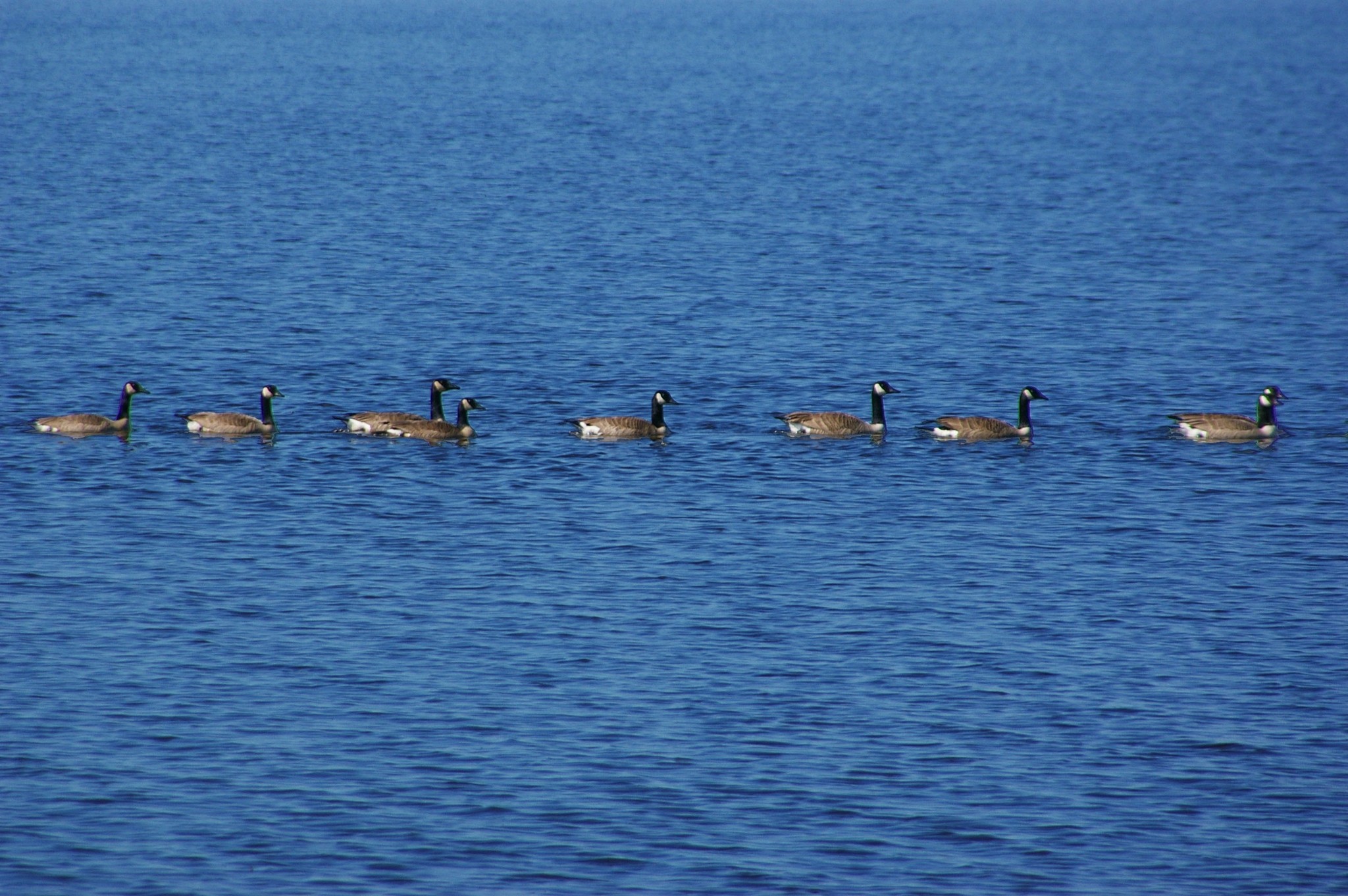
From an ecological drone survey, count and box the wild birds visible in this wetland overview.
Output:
[172,384,285,434]
[332,379,460,433]
[770,381,900,437]
[24,380,152,433]
[914,385,1050,441]
[371,397,487,439]
[1166,386,1290,441]
[563,390,680,437]
